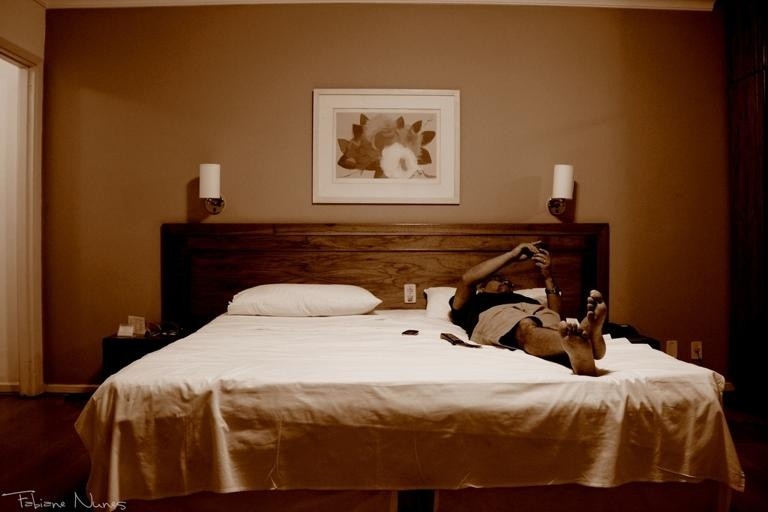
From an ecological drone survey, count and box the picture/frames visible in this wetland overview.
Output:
[311,89,460,204]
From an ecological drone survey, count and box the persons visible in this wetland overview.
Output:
[448,240,610,376]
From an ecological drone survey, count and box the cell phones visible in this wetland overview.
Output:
[523,241,546,258]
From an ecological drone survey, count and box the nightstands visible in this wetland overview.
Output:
[102,331,178,377]
[613,331,654,349]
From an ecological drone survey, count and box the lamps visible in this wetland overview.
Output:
[198,163,225,214]
[547,164,574,215]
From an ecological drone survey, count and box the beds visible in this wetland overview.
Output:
[74,223,744,512]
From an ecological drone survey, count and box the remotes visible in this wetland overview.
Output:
[441,332,464,346]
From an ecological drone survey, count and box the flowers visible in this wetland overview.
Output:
[336,112,437,180]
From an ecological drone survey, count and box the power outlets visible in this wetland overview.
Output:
[690,342,703,359]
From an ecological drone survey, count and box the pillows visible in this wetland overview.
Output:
[424,286,561,321]
[227,282,383,316]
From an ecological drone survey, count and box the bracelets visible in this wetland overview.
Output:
[544,288,562,296]
